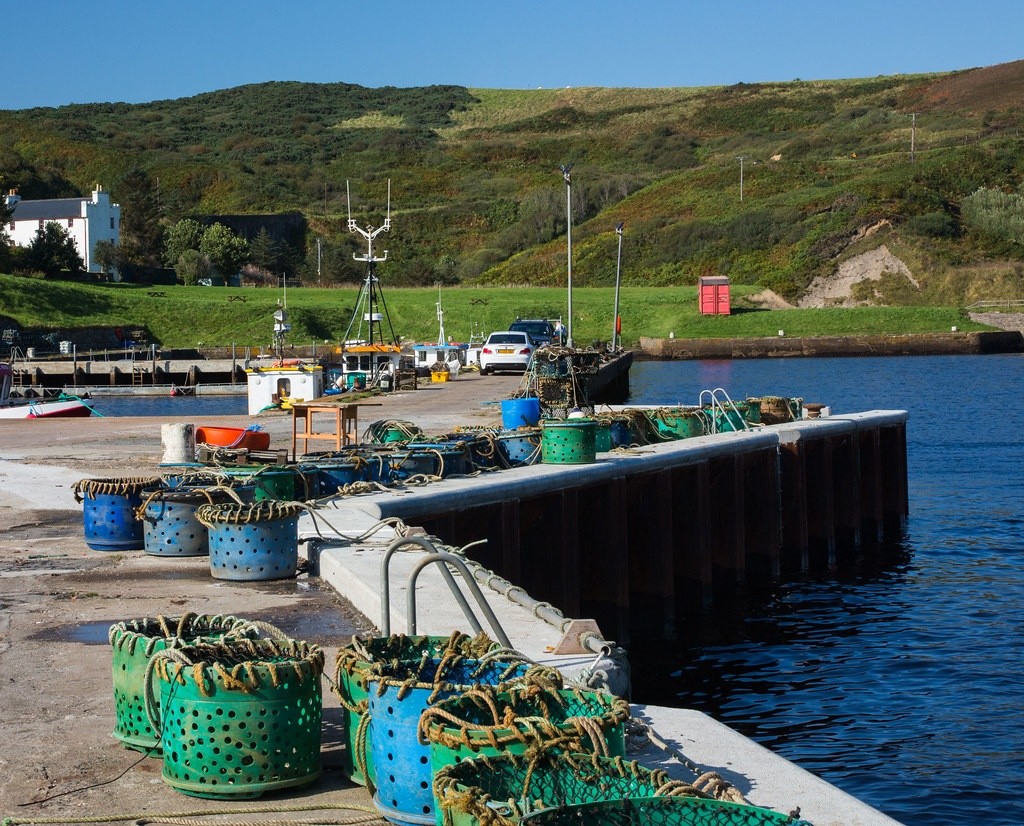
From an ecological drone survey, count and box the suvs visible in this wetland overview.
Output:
[509,315,556,348]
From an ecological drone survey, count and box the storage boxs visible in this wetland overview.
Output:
[431,371,451,383]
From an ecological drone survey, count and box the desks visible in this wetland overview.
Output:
[290,402,383,464]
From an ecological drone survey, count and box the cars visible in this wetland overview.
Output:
[480,332,539,377]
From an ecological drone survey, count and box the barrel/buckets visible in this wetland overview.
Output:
[502,398,538,430]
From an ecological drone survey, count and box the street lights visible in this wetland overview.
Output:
[559,162,573,352]
[611,220,627,354]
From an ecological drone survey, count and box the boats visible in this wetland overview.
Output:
[0,362,97,420]
[170,387,197,396]
